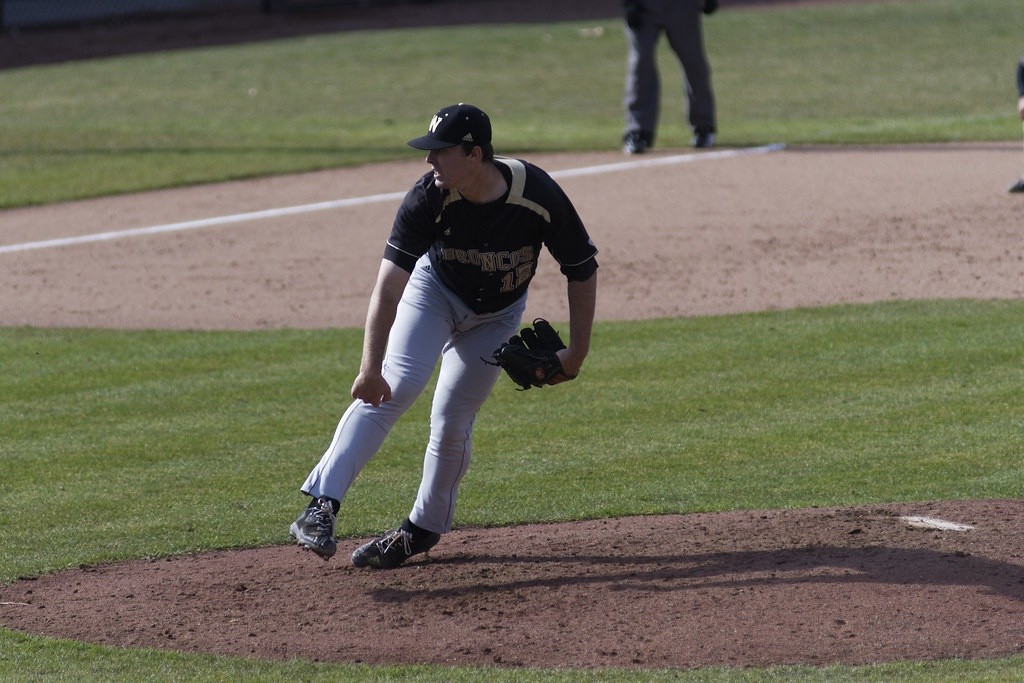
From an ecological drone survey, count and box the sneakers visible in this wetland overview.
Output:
[351,519,440,569]
[290,498,336,561]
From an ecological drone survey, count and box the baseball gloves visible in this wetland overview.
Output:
[478,316,581,393]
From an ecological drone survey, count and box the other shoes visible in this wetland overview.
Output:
[625,132,647,153]
[692,128,713,147]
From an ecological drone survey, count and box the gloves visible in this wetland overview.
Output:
[703,0,717,14]
[622,0,649,30]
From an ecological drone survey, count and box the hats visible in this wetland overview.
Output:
[407,103,491,150]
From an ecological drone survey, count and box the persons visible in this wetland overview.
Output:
[290,103,600,570]
[622,0,721,155]
